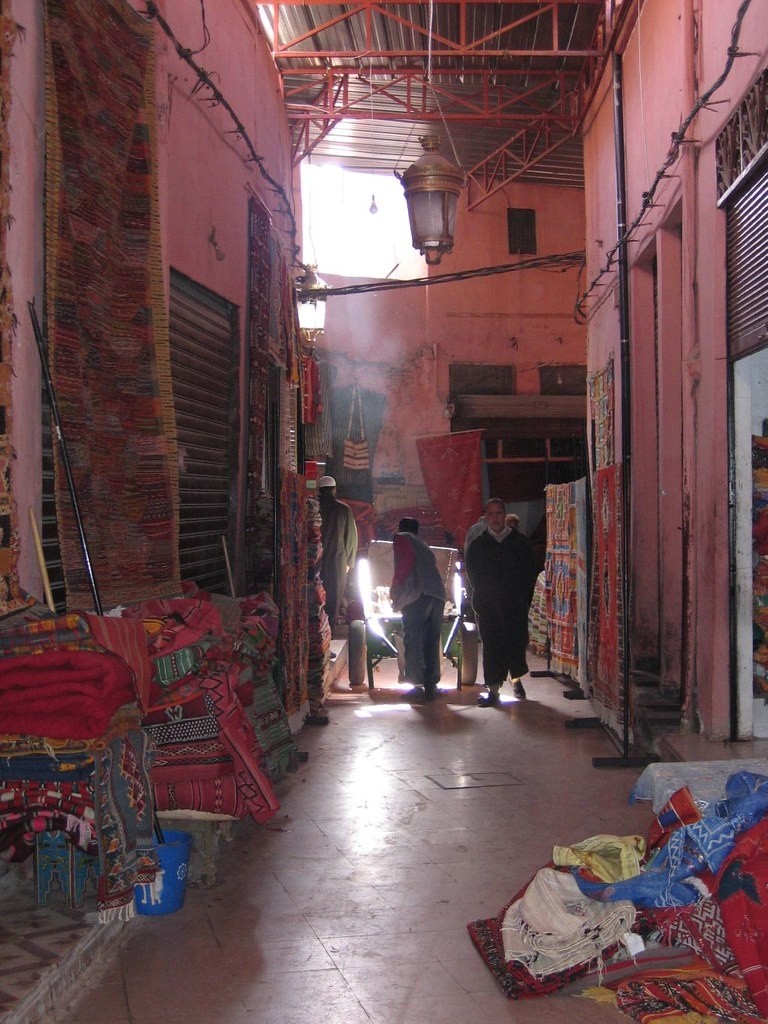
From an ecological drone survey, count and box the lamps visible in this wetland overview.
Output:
[393,136,468,267]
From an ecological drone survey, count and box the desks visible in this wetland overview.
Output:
[631,757,768,817]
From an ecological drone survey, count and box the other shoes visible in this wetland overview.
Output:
[513,682,526,698]
[330,652,336,658]
[477,692,500,705]
[400,687,424,698]
[425,684,447,697]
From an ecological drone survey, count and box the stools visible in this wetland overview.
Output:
[155,809,241,886]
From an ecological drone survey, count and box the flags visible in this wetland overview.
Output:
[416,431,483,549]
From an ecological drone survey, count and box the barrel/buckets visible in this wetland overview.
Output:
[132,831,191,914]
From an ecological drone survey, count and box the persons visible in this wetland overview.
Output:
[316,476,358,639]
[389,517,447,701]
[464,497,539,705]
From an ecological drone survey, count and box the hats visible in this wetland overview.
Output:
[318,476,336,487]
